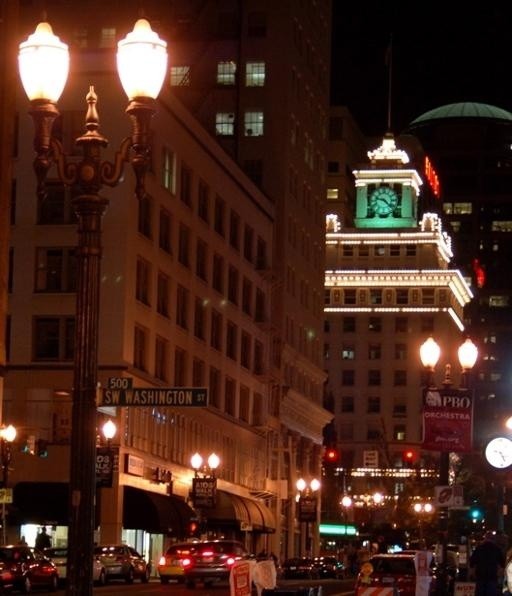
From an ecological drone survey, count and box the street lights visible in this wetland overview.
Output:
[19,17,166,595]
[417,336,477,596]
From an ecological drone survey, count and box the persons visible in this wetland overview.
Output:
[469,532,506,596]
[258,549,267,557]
[247,128,253,136]
[428,554,457,596]
[35,527,52,550]
[269,551,278,568]
[348,548,358,578]
[17,536,28,545]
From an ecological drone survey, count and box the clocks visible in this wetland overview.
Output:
[366,180,402,219]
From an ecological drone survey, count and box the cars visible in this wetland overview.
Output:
[283,554,346,580]
[354,550,434,595]
[157,538,281,590]
[1,545,59,594]
[93,544,151,585]
[43,548,107,587]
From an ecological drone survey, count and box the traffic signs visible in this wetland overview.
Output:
[102,387,209,408]
[364,450,378,468]
